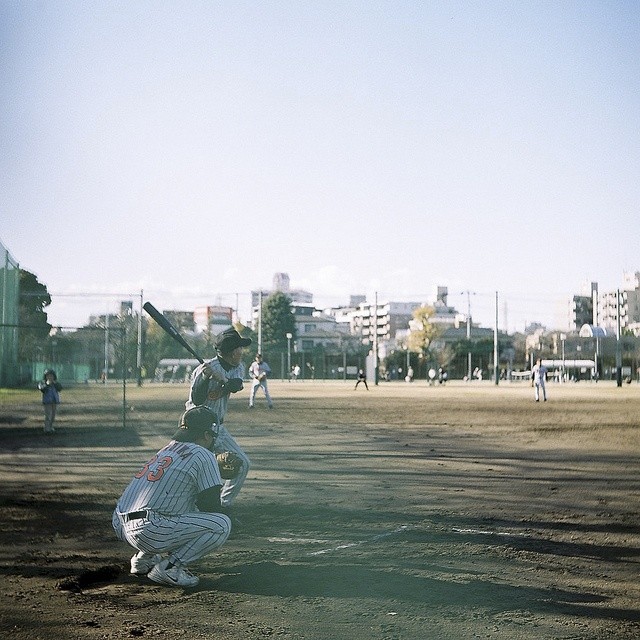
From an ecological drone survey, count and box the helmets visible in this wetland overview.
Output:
[44,369,57,380]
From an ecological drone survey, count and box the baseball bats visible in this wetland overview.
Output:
[142,302,203,364]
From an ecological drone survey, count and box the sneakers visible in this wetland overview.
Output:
[248,405,253,409]
[43,428,51,433]
[544,397,547,401]
[267,405,272,409]
[147,560,200,588]
[222,505,243,529]
[537,398,540,401]
[50,426,56,431]
[129,552,162,577]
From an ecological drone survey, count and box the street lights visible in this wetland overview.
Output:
[286,332,292,373]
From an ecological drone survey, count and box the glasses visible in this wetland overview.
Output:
[210,425,221,439]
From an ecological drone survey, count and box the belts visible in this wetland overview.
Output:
[122,510,148,521]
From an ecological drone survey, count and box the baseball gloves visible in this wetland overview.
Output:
[216,451,243,479]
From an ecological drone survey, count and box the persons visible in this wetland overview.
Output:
[290,363,303,382]
[111,404,242,586]
[185,328,253,508]
[355,368,370,392]
[530,358,548,401]
[37,368,62,434]
[247,353,274,408]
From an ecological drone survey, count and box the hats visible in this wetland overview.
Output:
[255,353,262,358]
[170,405,220,442]
[537,358,542,360]
[212,328,251,352]
